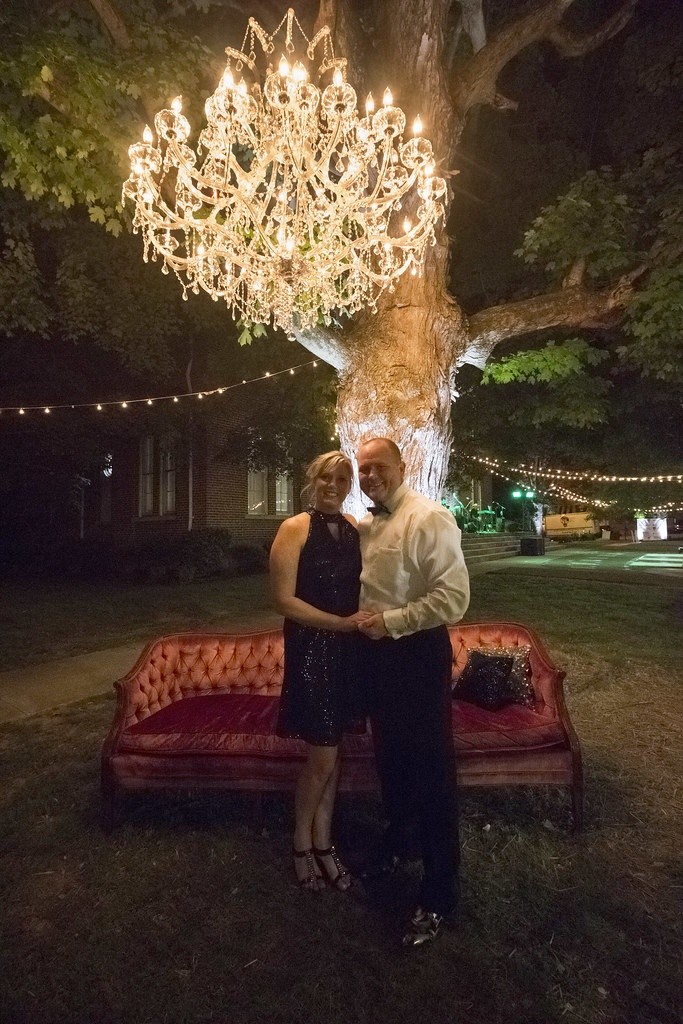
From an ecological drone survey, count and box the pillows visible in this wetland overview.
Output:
[453,651,515,710]
[464,644,537,711]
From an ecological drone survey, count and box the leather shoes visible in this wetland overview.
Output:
[396,903,448,947]
[359,846,419,884]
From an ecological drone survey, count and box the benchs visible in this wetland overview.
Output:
[98,618,585,832]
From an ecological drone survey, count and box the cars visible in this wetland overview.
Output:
[672,519,683,532]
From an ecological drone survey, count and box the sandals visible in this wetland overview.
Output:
[312,840,352,892]
[292,846,326,892]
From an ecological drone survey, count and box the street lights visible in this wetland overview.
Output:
[512,487,534,531]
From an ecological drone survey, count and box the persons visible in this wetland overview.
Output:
[355,437,471,951]
[268,451,370,894]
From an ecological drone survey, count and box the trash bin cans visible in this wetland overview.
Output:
[521,538,545,555]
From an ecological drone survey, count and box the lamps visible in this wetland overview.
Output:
[120,8,455,341]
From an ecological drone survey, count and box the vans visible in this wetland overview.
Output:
[544,505,601,538]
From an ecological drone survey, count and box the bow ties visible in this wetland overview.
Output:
[367,505,391,516]
